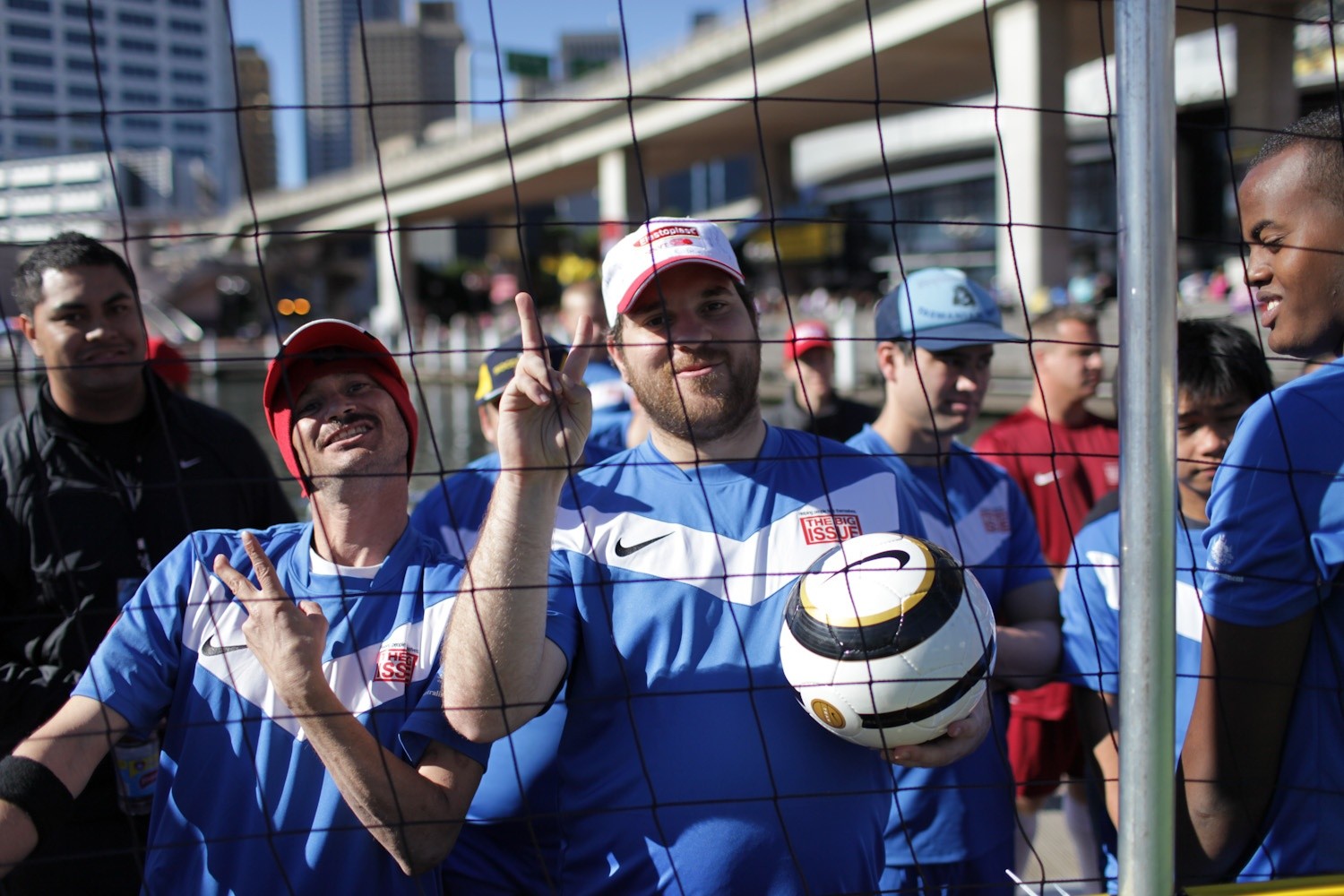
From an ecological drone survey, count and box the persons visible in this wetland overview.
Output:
[844,267,1064,896]
[972,303,1119,896]
[1179,264,1231,305]
[443,217,994,896]
[1058,319,1274,896]
[0,319,493,896]
[559,282,649,452]
[412,332,623,896]
[1177,110,1344,896]
[765,320,881,444]
[0,231,299,896]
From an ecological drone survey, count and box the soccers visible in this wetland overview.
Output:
[781,533,998,747]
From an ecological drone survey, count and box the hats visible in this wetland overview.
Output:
[263,317,410,442]
[874,268,1025,350]
[785,321,833,360]
[474,335,588,405]
[270,355,423,498]
[601,216,751,327]
